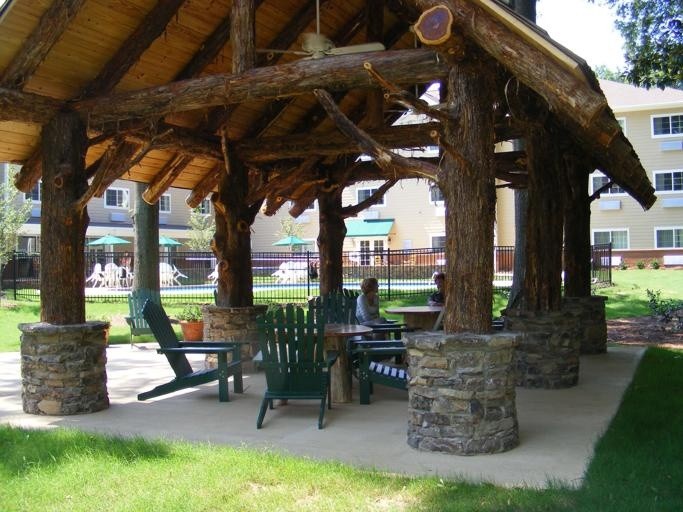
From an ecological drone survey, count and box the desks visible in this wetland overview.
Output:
[385,306,441,331]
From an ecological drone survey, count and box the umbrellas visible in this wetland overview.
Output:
[86,233,132,251]
[159,234,183,247]
[272,235,313,257]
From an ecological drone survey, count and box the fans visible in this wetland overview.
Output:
[256,0,386,60]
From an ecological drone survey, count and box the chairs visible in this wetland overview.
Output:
[86,262,122,290]
[207,264,218,284]
[125,287,161,347]
[138,298,249,401]
[256,288,421,429]
[171,264,189,286]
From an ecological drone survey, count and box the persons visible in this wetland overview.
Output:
[355,277,390,341]
[426,271,445,307]
[121,251,132,277]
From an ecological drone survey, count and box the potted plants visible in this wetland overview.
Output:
[175,305,203,340]
[650,258,660,269]
[636,260,645,269]
